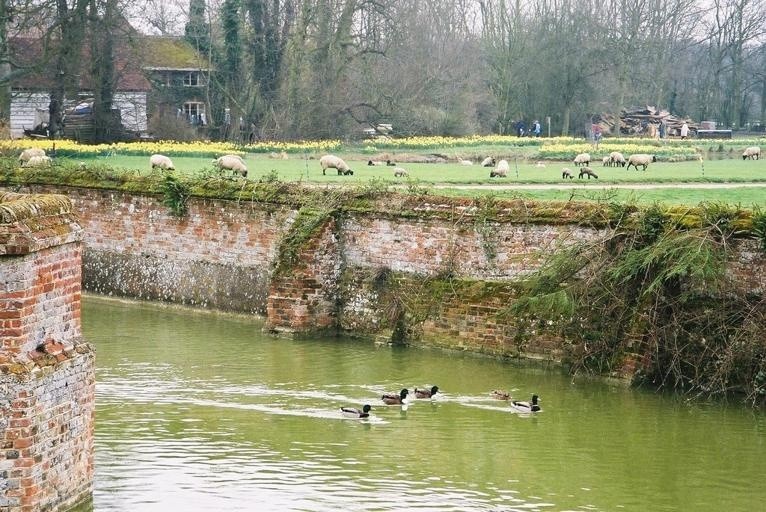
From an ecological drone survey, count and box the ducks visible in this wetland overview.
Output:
[340,404,376,418]
[491,389,510,400]
[511,393,542,412]
[414,386,441,398]
[382,389,409,405]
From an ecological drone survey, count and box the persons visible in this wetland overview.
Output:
[645,119,657,139]
[529,120,543,137]
[680,120,692,141]
[658,119,666,138]
[513,119,525,136]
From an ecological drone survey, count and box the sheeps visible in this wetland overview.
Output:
[562,168,575,180]
[579,167,598,179]
[218,155,248,177]
[481,157,509,177]
[386,160,396,166]
[18,148,53,167]
[457,158,472,166]
[603,152,657,171]
[573,153,591,167]
[151,155,175,175]
[742,146,760,160]
[320,155,353,175]
[392,168,409,177]
